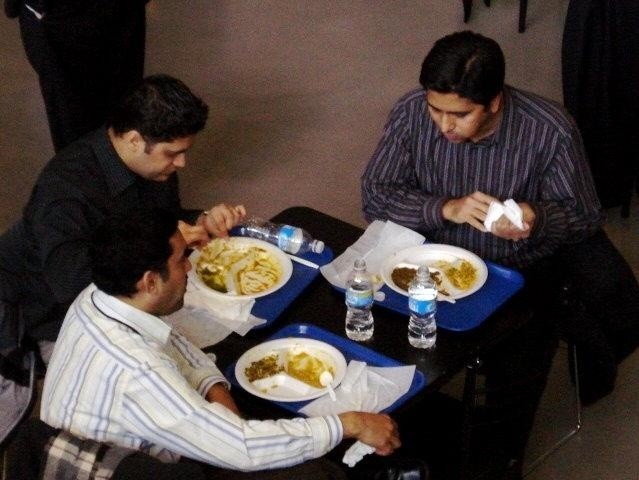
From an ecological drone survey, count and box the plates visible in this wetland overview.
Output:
[185,236,294,300]
[235,339,349,402]
[382,242,488,302]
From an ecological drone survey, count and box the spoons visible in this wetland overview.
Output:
[318,370,338,403]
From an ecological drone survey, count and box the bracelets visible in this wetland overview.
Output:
[202,209,208,229]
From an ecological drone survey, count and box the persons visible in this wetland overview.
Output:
[20,70,248,365]
[40,194,404,479]
[359,30,607,478]
[2,0,148,154]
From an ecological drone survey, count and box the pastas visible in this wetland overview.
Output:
[241,265,277,294]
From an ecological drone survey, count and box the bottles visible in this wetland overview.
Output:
[345,260,376,342]
[406,266,438,350]
[237,213,326,256]
